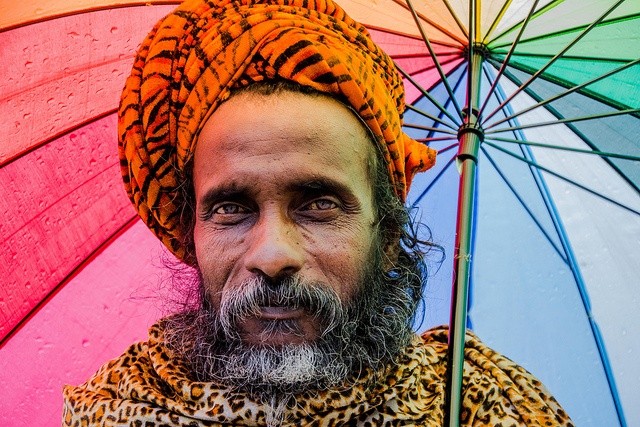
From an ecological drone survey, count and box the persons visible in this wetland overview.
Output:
[60,0,572,426]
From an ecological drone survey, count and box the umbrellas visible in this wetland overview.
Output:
[1,0,640,426]
